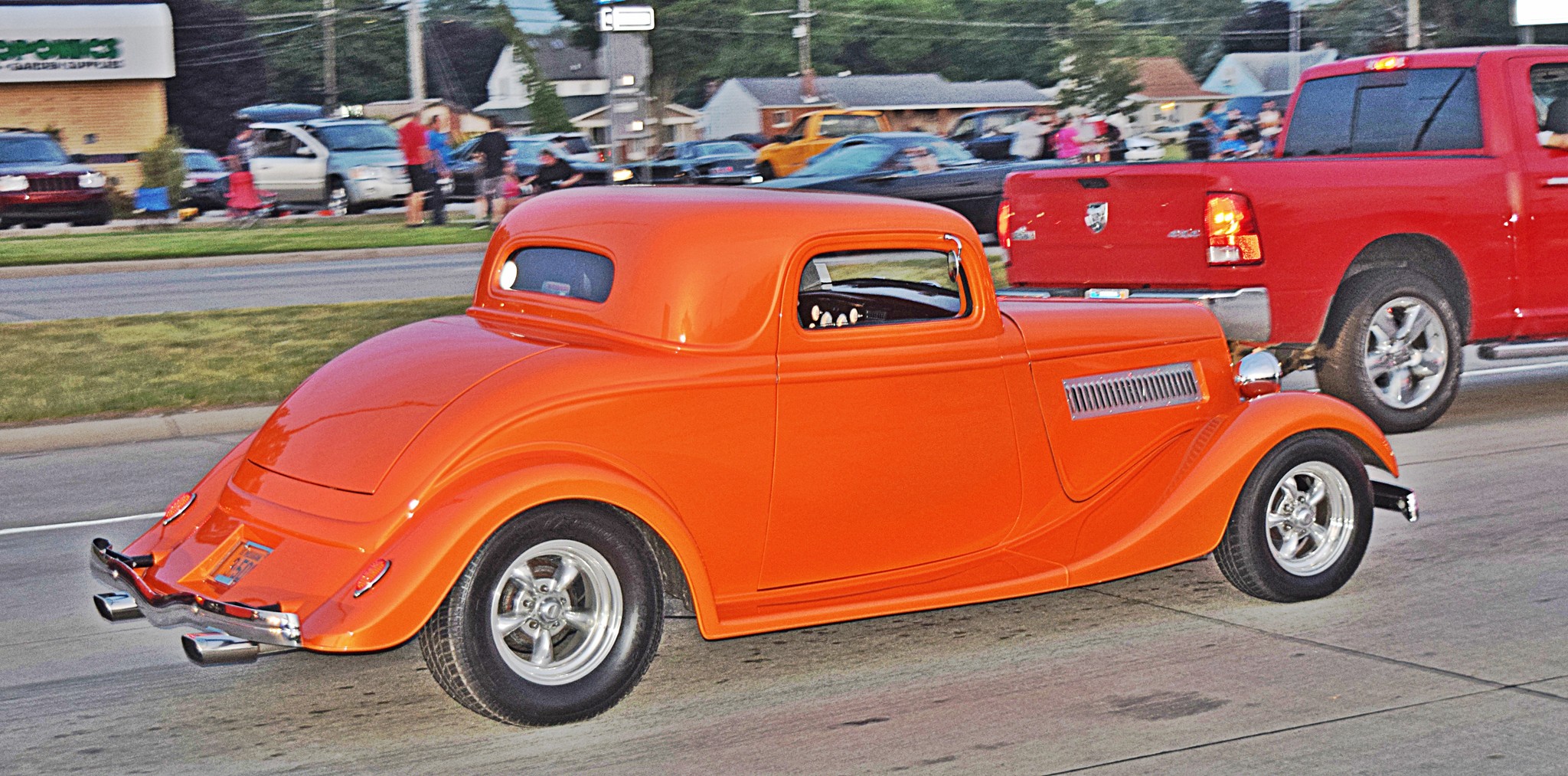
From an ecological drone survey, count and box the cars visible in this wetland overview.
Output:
[87,186,1421,731]
[0,132,111,228]
[739,131,1079,257]
[173,149,234,213]
[941,107,1205,161]
[626,131,759,187]
[755,108,900,179]
[438,130,636,191]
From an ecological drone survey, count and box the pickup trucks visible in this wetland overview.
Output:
[995,48,1567,435]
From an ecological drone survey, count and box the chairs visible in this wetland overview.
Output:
[133,187,171,220]
[225,171,276,218]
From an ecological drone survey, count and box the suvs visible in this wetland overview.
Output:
[231,104,456,214]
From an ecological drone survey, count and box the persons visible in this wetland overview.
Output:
[1187,99,1283,160]
[502,161,535,212]
[224,127,256,173]
[532,147,584,193]
[400,109,452,225]
[1000,109,1127,164]
[471,114,513,231]
[1539,130,1568,151]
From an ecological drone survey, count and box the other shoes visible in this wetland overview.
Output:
[470,221,490,230]
[405,220,425,227]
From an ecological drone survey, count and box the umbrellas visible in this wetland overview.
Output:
[231,101,325,122]
